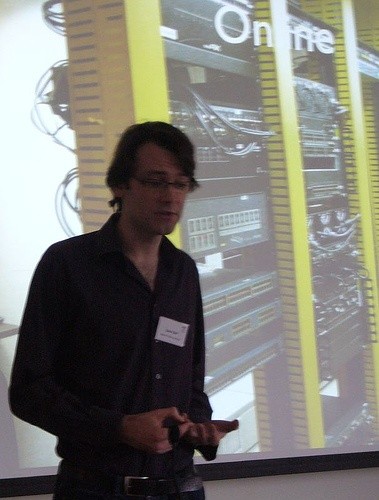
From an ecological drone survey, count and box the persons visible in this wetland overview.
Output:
[9,122,239,500]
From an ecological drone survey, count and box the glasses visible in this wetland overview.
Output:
[129,171,200,194]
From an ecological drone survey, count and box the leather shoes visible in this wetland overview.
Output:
[62,474,203,497]
